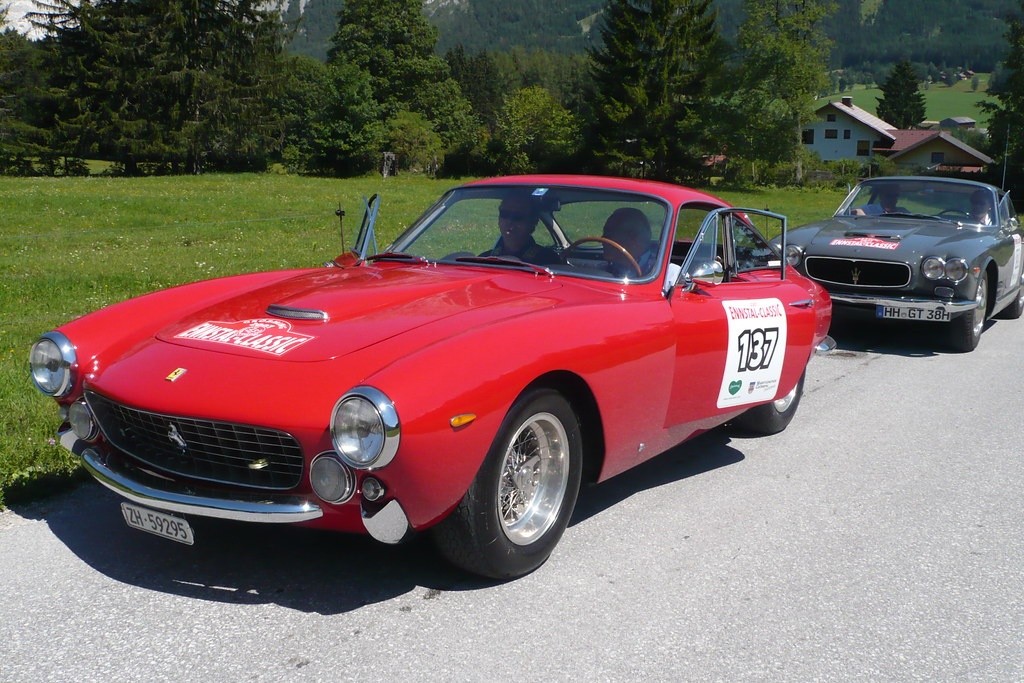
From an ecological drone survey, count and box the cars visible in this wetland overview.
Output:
[31,172,834,580]
[764,175,1022,354]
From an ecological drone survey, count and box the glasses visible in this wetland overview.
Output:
[500,209,534,221]
[972,199,991,206]
[883,197,896,204]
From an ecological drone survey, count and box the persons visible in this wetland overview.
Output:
[851,184,911,215]
[601,207,690,296]
[971,190,992,225]
[478,199,559,266]
